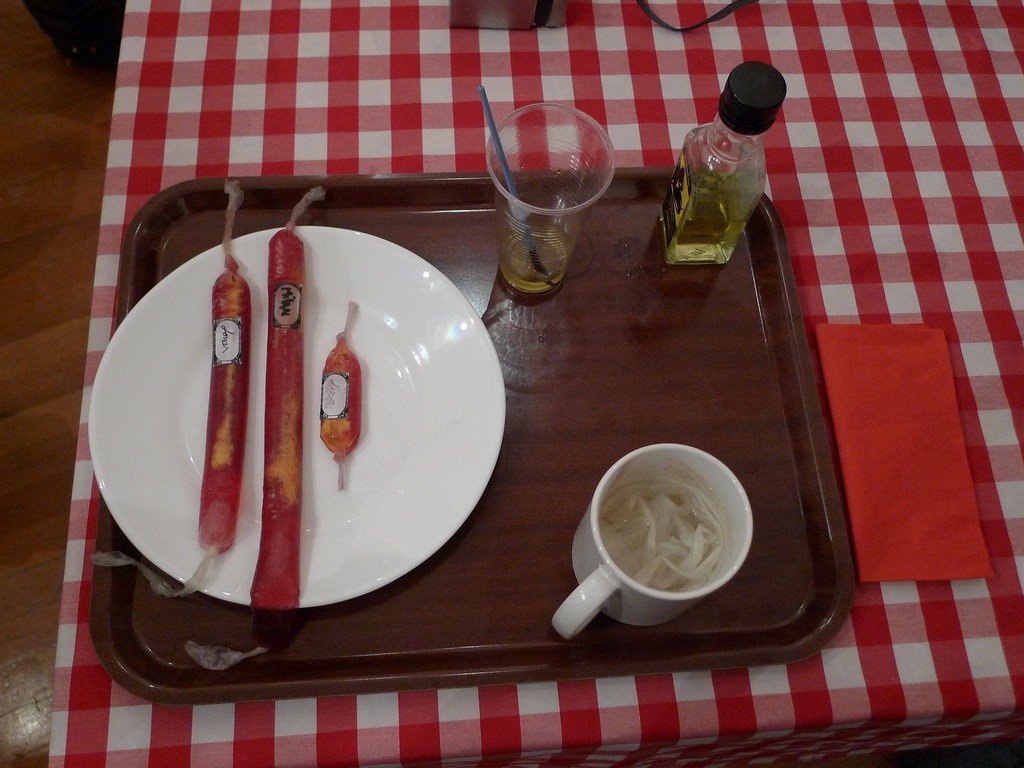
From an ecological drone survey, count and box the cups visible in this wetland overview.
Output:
[485,103,617,295]
[551,442,754,642]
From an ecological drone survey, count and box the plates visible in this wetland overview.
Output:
[86,226,507,610]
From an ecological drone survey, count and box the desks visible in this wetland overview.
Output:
[49,0,1024,768]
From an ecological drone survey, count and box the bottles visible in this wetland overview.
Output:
[659,61,788,266]
